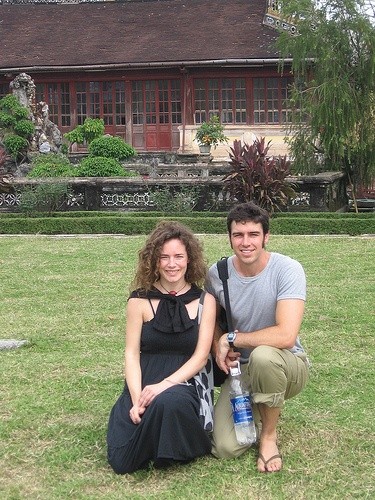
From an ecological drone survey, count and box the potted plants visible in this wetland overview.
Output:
[193,113,229,156]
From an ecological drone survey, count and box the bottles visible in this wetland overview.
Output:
[230,368,253,446]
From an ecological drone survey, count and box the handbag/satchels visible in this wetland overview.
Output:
[210,257,231,387]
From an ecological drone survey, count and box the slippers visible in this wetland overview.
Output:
[257,453,282,472]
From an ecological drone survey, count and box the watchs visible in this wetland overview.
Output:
[226,332,237,348]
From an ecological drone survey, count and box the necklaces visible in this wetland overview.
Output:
[160,280,186,295]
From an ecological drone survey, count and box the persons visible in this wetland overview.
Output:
[203,201,308,471]
[108,220,216,475]
